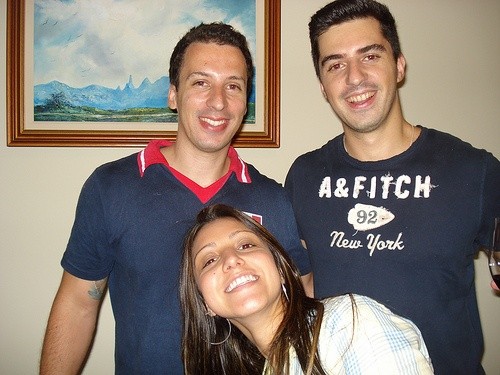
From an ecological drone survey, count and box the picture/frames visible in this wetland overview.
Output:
[6,0,281,149]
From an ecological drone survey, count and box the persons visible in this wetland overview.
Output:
[43,22,315,375]
[284,0,500,375]
[181,203,435,375]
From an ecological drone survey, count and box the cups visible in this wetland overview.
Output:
[488,218,500,290]
